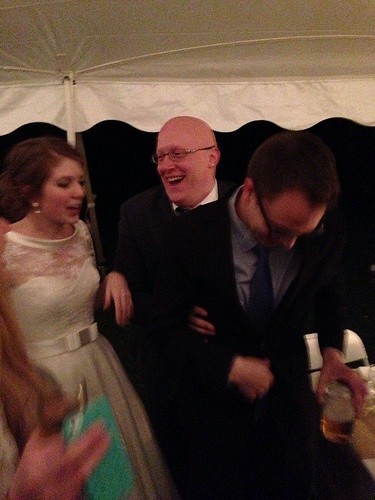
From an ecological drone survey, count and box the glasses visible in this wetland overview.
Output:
[254,189,323,243]
[152,145,217,164]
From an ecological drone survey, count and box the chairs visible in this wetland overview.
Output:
[302,328,370,395]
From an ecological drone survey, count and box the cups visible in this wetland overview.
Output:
[320,382,355,445]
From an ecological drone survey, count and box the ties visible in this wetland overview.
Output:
[249,246,277,332]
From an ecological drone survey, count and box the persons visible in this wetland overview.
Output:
[0,136,173,500]
[95,115,375,500]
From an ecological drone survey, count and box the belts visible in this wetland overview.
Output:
[24,321,98,360]
